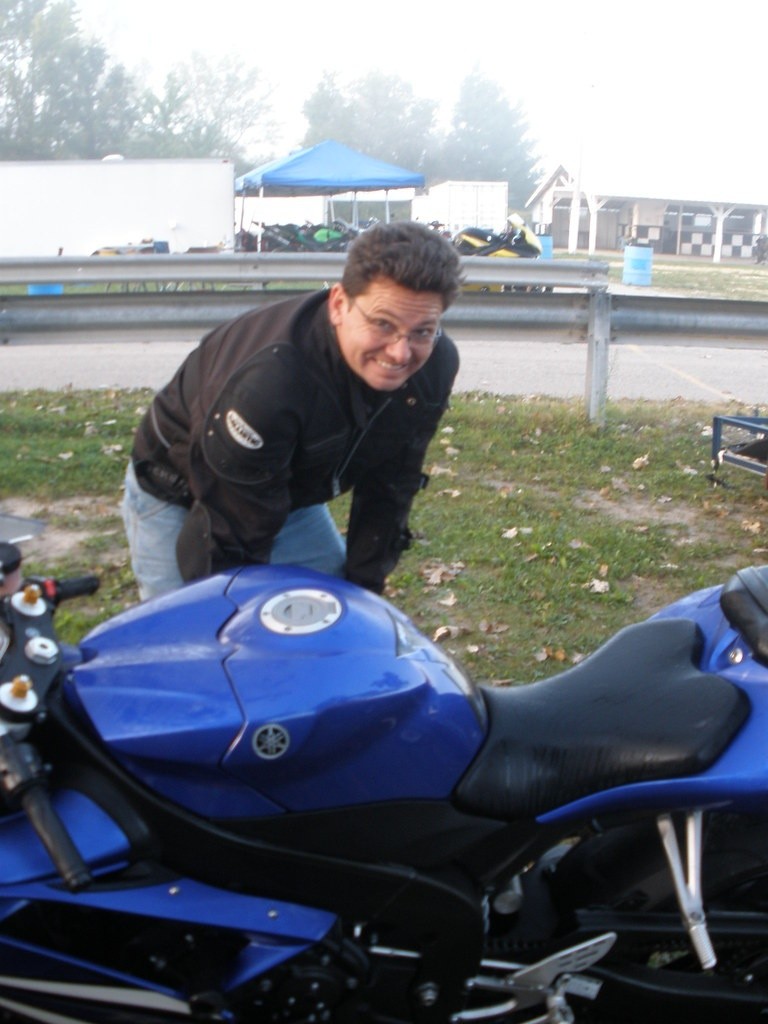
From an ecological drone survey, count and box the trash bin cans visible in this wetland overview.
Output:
[621,245,654,286]
[537,235,554,258]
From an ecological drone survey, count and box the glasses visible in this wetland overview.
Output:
[347,292,442,349]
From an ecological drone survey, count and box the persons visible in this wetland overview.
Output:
[121,221,460,602]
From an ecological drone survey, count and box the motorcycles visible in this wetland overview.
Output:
[0,519,768,1024]
[447,213,554,295]
[240,210,452,256]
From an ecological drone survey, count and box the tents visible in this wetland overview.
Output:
[235,140,425,254]
